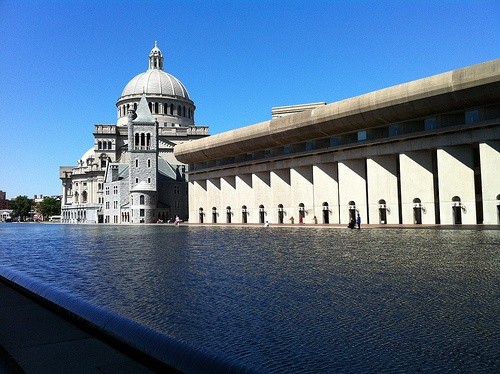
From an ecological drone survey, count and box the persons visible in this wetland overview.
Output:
[265,221,269,227]
[290,217,294,223]
[299,212,302,223]
[348,218,355,228]
[357,213,362,229]
[175,215,180,226]
[312,215,317,223]
